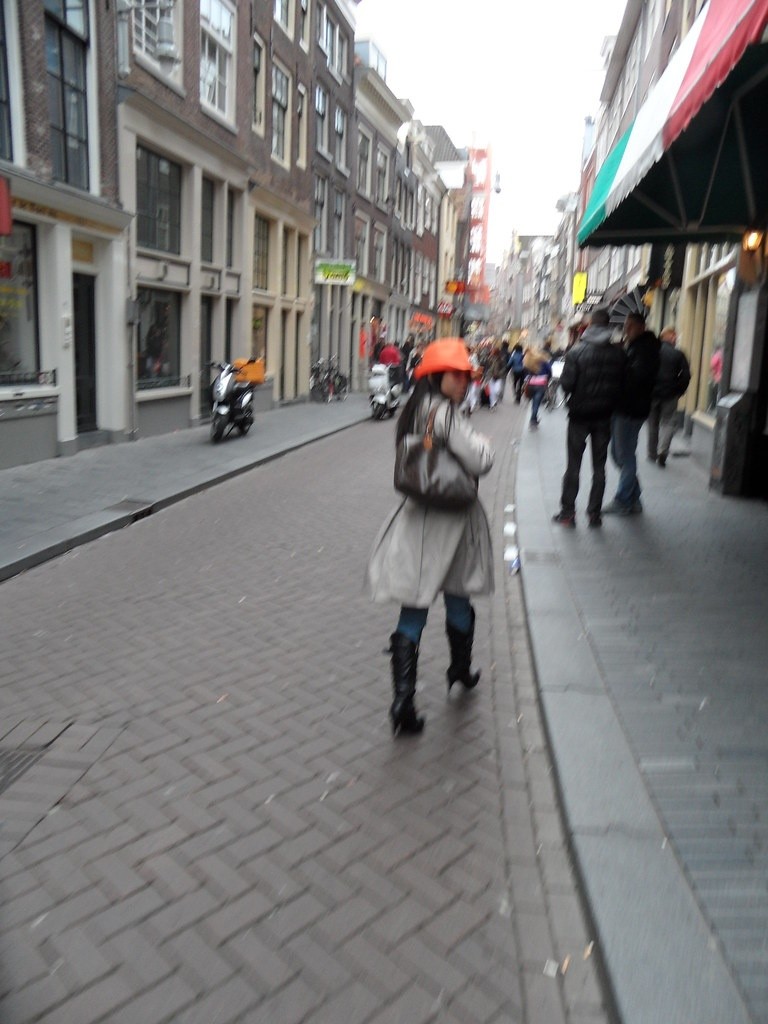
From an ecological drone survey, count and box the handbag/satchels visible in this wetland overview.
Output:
[529,374,548,386]
[394,401,479,508]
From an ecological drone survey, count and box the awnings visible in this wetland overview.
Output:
[575,0,768,248]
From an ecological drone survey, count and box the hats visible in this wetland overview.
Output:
[414,338,482,380]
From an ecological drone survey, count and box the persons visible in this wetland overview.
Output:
[603,313,660,514]
[708,346,723,410]
[372,338,557,426]
[367,336,493,736]
[647,327,691,466]
[549,310,617,527]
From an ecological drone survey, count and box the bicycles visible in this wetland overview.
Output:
[308,353,350,406]
[535,373,567,424]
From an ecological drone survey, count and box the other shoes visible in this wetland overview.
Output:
[552,511,575,529]
[591,517,601,526]
[601,497,642,516]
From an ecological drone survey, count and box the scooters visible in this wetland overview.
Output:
[204,356,260,443]
[368,360,405,421]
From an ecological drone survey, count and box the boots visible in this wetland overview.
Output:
[386,632,423,733]
[446,629,479,690]
